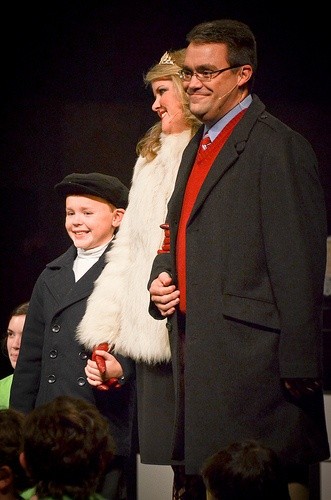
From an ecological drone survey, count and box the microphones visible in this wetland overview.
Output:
[218,76,243,100]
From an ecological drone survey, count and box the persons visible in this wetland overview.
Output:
[147,19,331,500]
[76,47,202,500]
[9,173,136,500]
[0,303,331,500]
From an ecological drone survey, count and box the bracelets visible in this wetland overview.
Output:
[120,376,125,385]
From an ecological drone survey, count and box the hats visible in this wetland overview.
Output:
[51,171,129,208]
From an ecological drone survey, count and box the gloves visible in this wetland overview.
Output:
[92,343,121,389]
[157,224,171,254]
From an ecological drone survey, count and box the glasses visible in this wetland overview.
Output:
[177,66,234,81]
